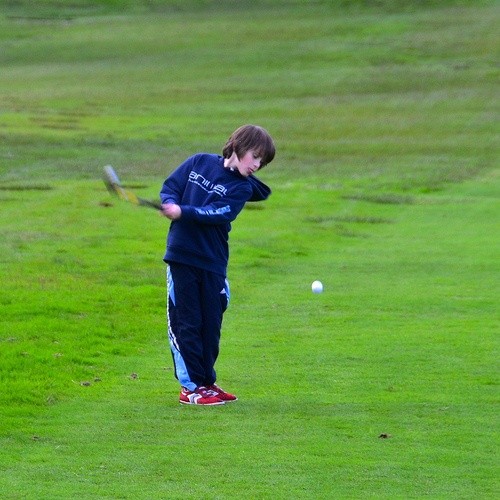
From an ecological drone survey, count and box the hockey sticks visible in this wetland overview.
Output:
[100,164,165,210]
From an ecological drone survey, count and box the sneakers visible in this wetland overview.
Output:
[179,386,225,405]
[203,384,238,402]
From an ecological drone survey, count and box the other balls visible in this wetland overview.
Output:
[312,280,323,294]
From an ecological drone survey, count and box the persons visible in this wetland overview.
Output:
[160,124,276,407]
